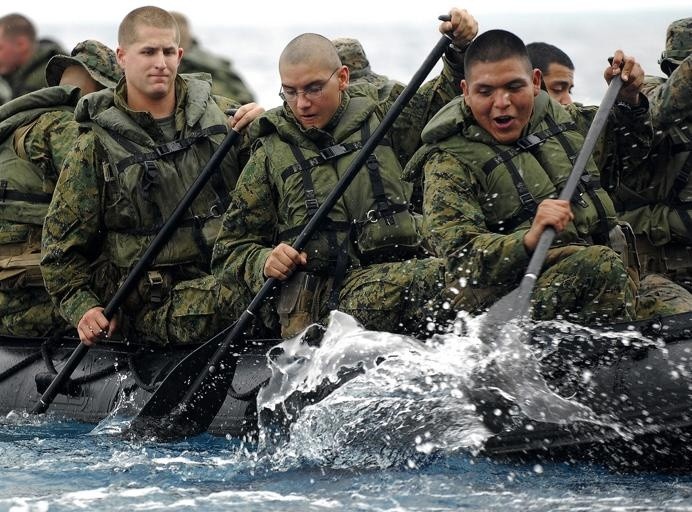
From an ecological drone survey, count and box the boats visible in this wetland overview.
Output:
[0,307,691,474]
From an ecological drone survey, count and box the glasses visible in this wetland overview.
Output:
[279,66,339,102]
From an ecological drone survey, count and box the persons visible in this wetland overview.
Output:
[2,7,480,347]
[403,17,692,326]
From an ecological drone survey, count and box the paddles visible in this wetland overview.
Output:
[120,15,455,439]
[480,56,625,343]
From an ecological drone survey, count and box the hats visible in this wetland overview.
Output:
[45,39,122,89]
[659,18,691,76]
[333,38,387,93]
